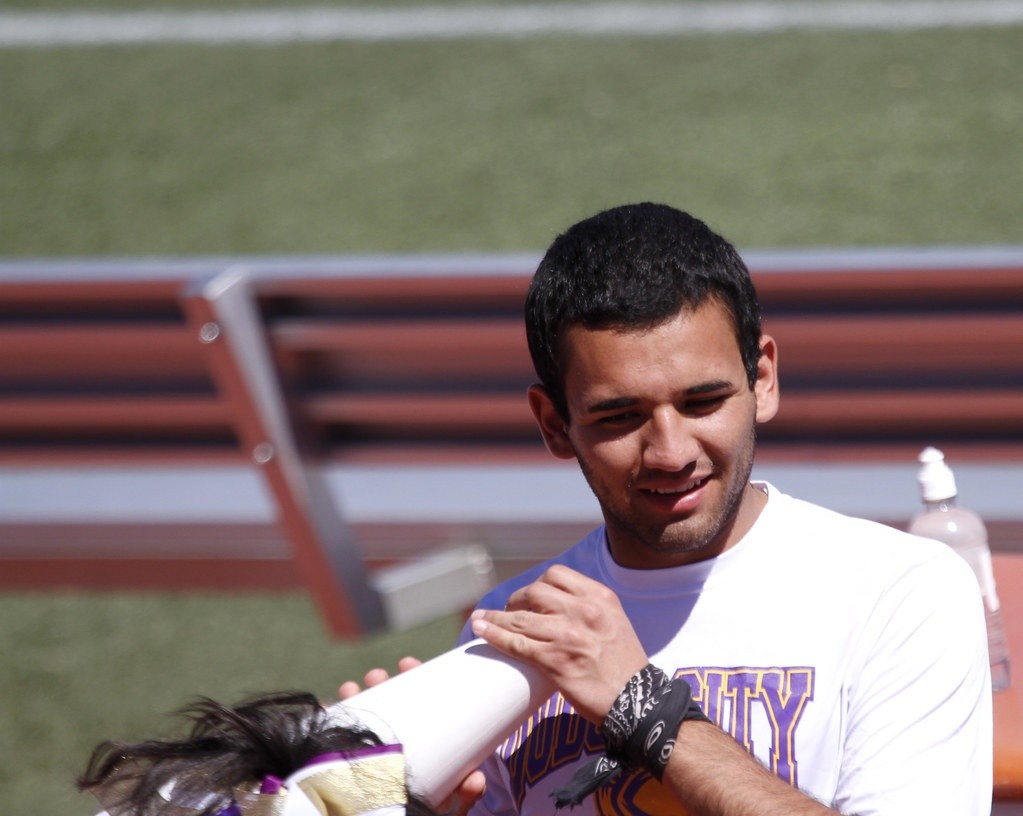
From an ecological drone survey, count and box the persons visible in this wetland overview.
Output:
[905,445,1003,621]
[71,685,440,816]
[312,199,997,816]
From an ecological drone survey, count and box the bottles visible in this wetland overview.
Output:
[906,448,1015,695]
